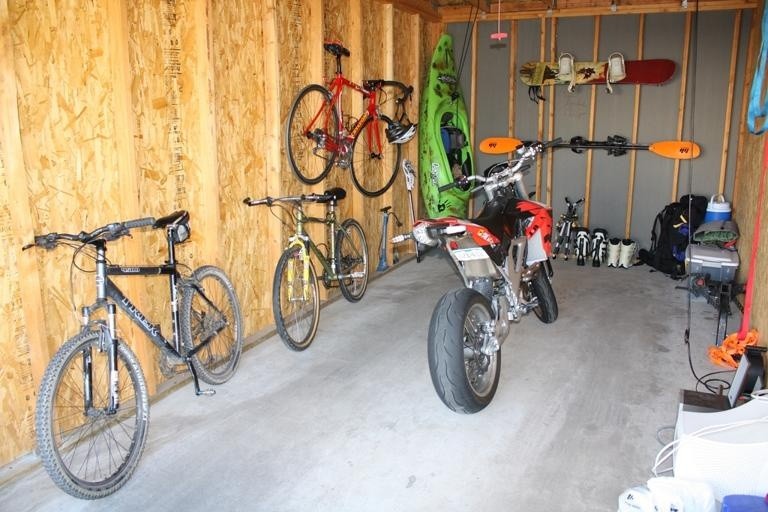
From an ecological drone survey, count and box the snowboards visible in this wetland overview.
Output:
[519,54,675,93]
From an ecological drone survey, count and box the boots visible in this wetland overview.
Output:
[606,238,618,268]
[591,229,605,267]
[574,226,589,266]
[618,238,636,270]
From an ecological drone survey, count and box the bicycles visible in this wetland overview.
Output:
[21,210,244,501]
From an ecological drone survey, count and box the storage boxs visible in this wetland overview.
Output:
[684,240,740,286]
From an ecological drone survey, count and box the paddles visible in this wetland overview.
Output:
[479,137,700,160]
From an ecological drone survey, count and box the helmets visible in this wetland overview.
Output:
[385,123,415,144]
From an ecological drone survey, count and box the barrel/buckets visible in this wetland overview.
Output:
[704,192,733,223]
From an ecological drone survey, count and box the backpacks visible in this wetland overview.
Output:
[638,201,696,277]
